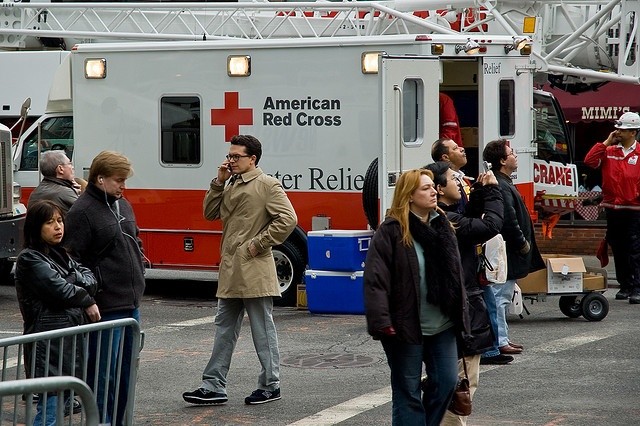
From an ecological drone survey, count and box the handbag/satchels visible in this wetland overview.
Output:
[485,234,510,284]
[422,355,473,426]
[458,287,496,349]
[507,283,523,317]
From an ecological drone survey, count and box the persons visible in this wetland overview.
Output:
[21,151,85,404]
[182,135,298,405]
[421,161,506,425]
[580,109,640,304]
[67,150,144,425]
[431,140,514,365]
[578,173,603,194]
[13,199,103,425]
[482,140,549,357]
[438,92,463,148]
[361,167,471,425]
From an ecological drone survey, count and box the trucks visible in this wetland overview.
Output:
[533,86,580,207]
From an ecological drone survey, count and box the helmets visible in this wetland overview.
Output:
[614,112,640,129]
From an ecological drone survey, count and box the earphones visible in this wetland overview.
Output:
[100,177,103,182]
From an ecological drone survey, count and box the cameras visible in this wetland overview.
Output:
[481,160,493,173]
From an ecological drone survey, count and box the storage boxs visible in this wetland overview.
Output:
[516,252,585,295]
[304,230,374,272]
[304,269,364,315]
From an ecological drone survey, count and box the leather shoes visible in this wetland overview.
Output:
[500,343,523,353]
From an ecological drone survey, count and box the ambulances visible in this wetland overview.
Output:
[11,32,539,306]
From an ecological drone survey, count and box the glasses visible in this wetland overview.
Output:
[55,163,76,168]
[226,154,252,162]
[507,148,514,157]
[447,176,457,183]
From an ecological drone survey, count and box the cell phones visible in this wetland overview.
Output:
[228,163,232,171]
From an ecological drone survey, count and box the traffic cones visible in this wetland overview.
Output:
[296,284,309,309]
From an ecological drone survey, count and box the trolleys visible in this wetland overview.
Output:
[513,264,610,321]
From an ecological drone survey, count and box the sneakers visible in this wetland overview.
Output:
[480,354,513,364]
[630,288,640,304]
[244,388,281,405]
[181,388,228,405]
[616,289,630,298]
